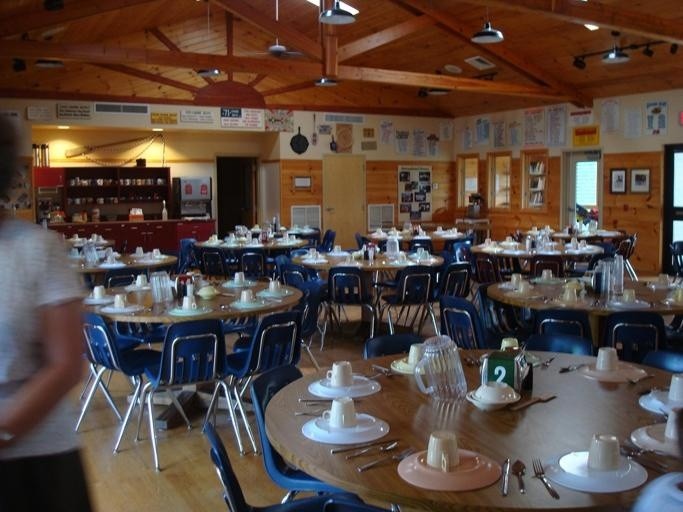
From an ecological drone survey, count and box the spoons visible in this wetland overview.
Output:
[343,439,399,459]
[510,459,527,497]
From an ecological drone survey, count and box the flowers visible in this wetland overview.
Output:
[469,193,483,204]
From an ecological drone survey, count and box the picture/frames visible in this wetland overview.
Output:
[630,167,650,194]
[610,168,626,193]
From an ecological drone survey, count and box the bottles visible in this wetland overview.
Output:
[120,191,166,201]
[162,200,167,221]
[121,177,166,185]
[71,196,119,205]
[71,177,115,185]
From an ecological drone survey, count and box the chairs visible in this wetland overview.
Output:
[295,279,322,372]
[642,348,682,374]
[275,255,290,280]
[354,232,371,250]
[604,229,640,282]
[529,307,595,339]
[530,254,564,278]
[378,265,440,338]
[362,334,424,362]
[104,267,136,287]
[377,239,403,252]
[452,241,477,274]
[238,248,266,280]
[76,310,192,434]
[232,308,306,458]
[179,237,202,275]
[408,239,433,253]
[202,420,364,512]
[600,311,667,356]
[310,227,336,253]
[524,333,594,357]
[290,249,310,256]
[440,295,523,351]
[201,247,230,277]
[281,265,308,287]
[248,363,359,504]
[669,241,683,284]
[136,317,246,474]
[477,258,497,284]
[321,266,375,348]
[420,261,473,336]
[587,243,615,276]
[510,229,523,242]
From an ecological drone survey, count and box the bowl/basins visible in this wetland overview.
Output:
[198,285,220,294]
[198,295,218,300]
[465,389,509,413]
[472,381,522,404]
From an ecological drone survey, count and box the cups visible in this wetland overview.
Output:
[114,294,127,309]
[326,360,354,387]
[595,347,620,370]
[108,255,116,265]
[676,288,683,302]
[413,334,468,404]
[407,343,429,366]
[417,248,424,256]
[421,251,430,260]
[148,269,174,304]
[322,395,357,428]
[135,247,144,255]
[484,239,491,247]
[89,286,105,299]
[132,274,148,287]
[426,429,461,473]
[241,289,253,303]
[500,338,520,351]
[580,240,586,248]
[668,371,682,403]
[622,288,636,304]
[71,248,78,256]
[563,286,578,304]
[334,245,341,254]
[541,269,553,283]
[269,281,281,292]
[181,295,197,310]
[517,281,530,293]
[345,255,354,265]
[510,274,522,288]
[658,273,669,286]
[665,407,682,439]
[397,252,407,262]
[152,248,161,259]
[587,435,622,472]
[234,271,245,285]
[309,248,317,260]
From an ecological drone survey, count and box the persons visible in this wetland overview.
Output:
[1,112,93,512]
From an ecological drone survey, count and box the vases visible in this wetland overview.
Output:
[473,205,481,219]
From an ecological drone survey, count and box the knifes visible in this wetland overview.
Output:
[500,457,511,497]
[329,437,391,453]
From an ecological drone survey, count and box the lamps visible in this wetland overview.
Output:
[315,77,337,87]
[427,88,448,96]
[197,69,219,77]
[470,22,504,44]
[601,48,630,64]
[33,59,64,69]
[318,1,355,24]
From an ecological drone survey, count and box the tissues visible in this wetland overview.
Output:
[128,208,144,222]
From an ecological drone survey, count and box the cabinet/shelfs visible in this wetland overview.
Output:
[521,148,548,213]
[34,166,173,224]
[48,218,216,253]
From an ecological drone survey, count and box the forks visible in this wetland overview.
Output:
[528,455,562,501]
[357,445,419,471]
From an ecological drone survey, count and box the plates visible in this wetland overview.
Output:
[300,413,391,446]
[306,374,383,399]
[630,423,683,458]
[395,449,504,493]
[545,451,651,496]
[123,284,152,291]
[389,357,425,376]
[255,288,294,299]
[229,300,265,309]
[583,365,648,383]
[303,259,329,264]
[99,303,146,314]
[83,294,114,306]
[221,280,258,288]
[393,261,414,266]
[328,252,349,256]
[167,306,214,316]
[638,391,669,416]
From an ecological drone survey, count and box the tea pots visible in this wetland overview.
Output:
[79,242,99,266]
